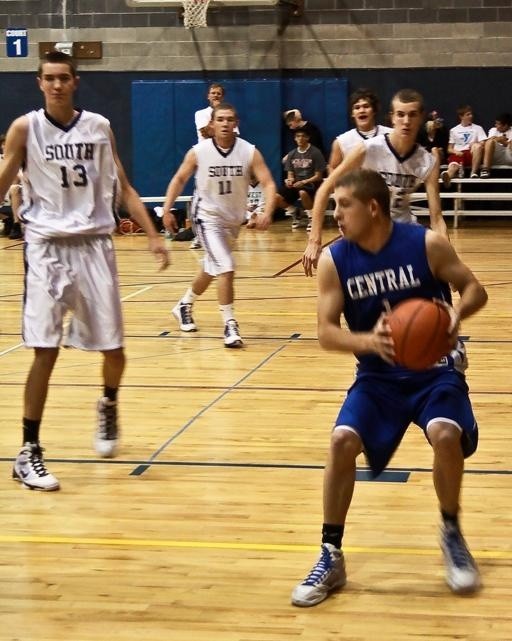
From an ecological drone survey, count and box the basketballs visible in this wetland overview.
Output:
[384,300,458,372]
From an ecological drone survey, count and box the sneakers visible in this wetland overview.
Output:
[440,170,452,189]
[288,543,348,610]
[290,206,304,231]
[1,217,25,240]
[170,299,199,333]
[222,318,244,350]
[469,171,479,179]
[305,217,313,232]
[93,396,120,460]
[435,519,484,597]
[479,169,491,179]
[12,441,62,494]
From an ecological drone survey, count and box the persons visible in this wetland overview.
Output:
[243,173,267,228]
[1,52,170,491]
[301,89,458,294]
[163,104,277,347]
[479,111,512,177]
[328,88,394,177]
[1,133,24,240]
[241,108,326,231]
[291,167,488,607]
[417,110,450,164]
[184,85,240,247]
[441,104,489,188]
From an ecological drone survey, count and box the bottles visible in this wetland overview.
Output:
[165,229,172,239]
[184,218,191,230]
[457,162,465,179]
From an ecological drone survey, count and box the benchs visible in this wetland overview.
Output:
[137,196,193,219]
[306,164,512,228]
